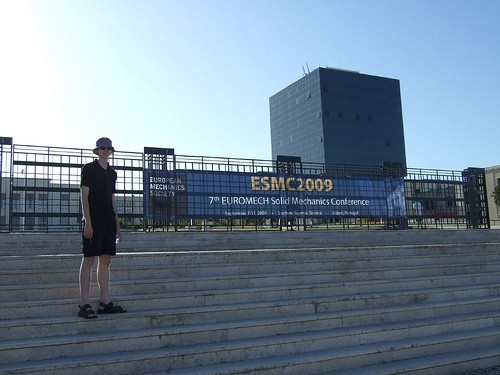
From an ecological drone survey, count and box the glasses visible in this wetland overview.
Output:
[98,147,112,151]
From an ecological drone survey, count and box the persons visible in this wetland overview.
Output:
[412,199,426,229]
[76,137,131,319]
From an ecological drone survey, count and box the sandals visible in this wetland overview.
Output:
[78,304,97,319]
[97,302,126,314]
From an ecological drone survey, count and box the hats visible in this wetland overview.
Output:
[93,137,114,155]
